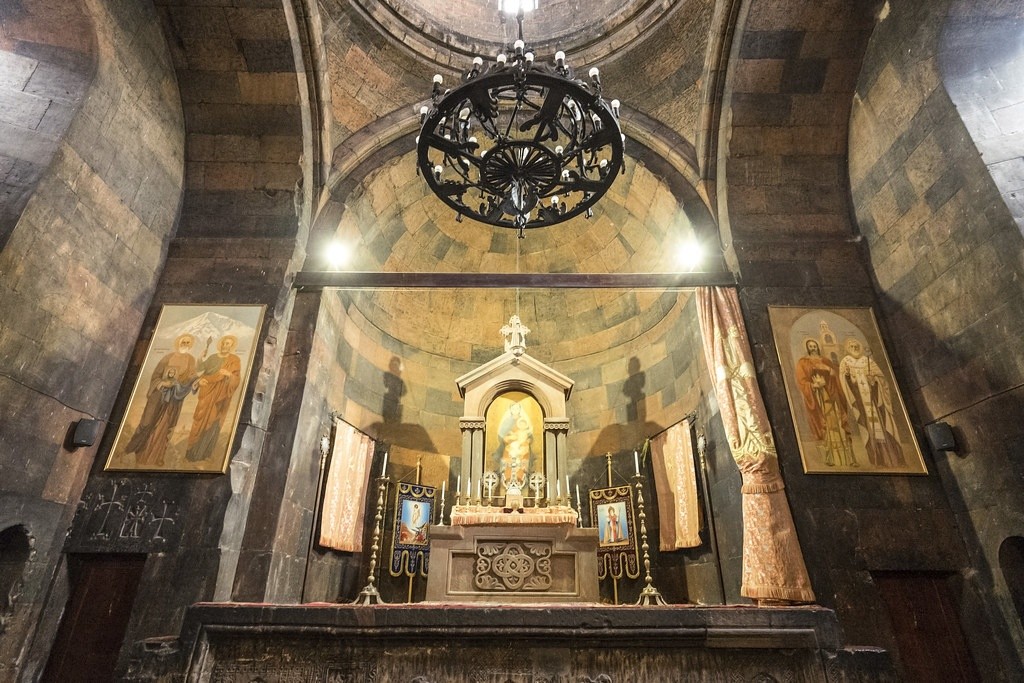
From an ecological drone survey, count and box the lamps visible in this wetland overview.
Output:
[411,1,628,240]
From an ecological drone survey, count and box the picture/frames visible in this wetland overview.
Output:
[766,299,933,479]
[398,497,431,548]
[102,298,267,476]
[481,391,549,505]
[594,499,632,549]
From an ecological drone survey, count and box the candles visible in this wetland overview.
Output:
[441,473,582,507]
[633,450,641,476]
[381,451,388,476]
